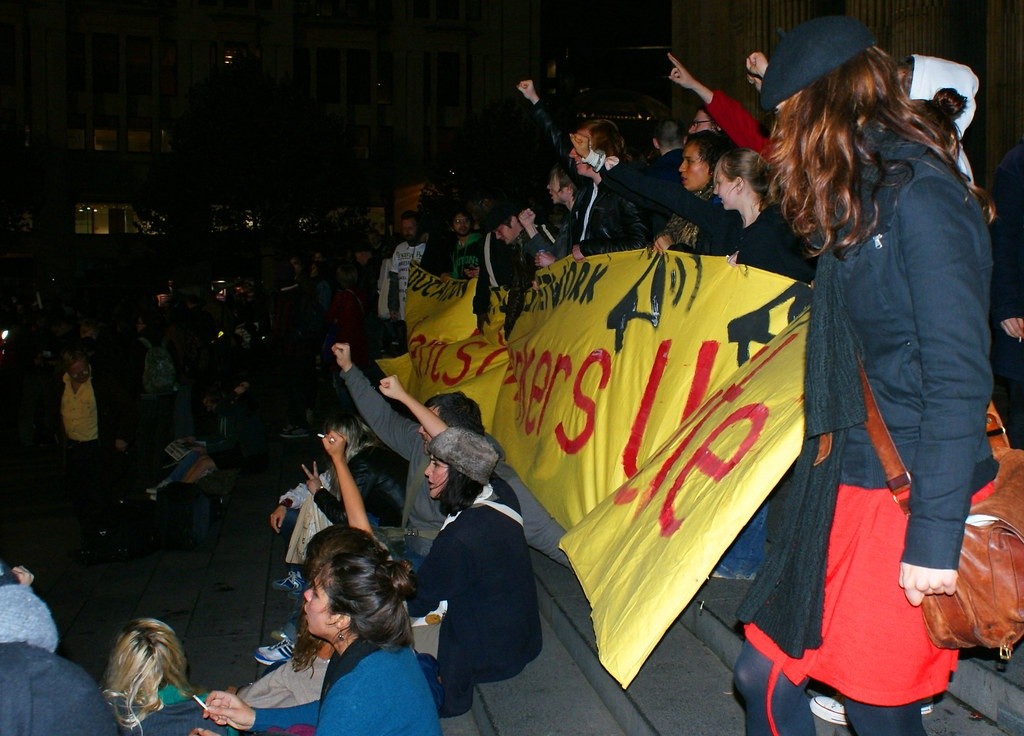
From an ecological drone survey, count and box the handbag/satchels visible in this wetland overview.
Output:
[921,451,1024,657]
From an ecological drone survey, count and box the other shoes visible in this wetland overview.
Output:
[145,480,167,502]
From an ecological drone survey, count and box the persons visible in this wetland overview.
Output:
[397,428,544,685]
[732,15,993,736]
[0,12,980,662]
[186,555,444,736]
[100,616,223,736]
[2,580,118,736]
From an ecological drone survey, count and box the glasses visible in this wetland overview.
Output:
[692,120,713,127]
[453,218,471,224]
[72,367,90,380]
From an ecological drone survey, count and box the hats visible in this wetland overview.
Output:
[759,14,877,113]
[427,425,499,487]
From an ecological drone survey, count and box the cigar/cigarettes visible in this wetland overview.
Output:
[192,694,214,718]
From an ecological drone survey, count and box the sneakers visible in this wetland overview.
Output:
[278,422,309,437]
[810,696,853,726]
[271,571,304,589]
[252,639,296,666]
[271,630,291,641]
[920,695,935,715]
